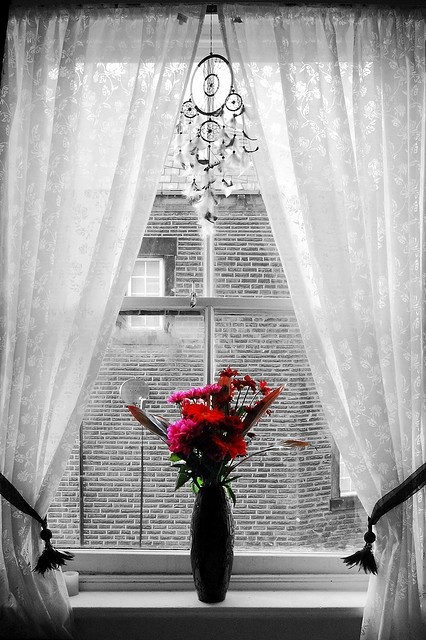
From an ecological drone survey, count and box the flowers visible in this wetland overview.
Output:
[126,365,318,507]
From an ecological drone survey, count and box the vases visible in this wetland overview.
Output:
[190,486,234,603]
